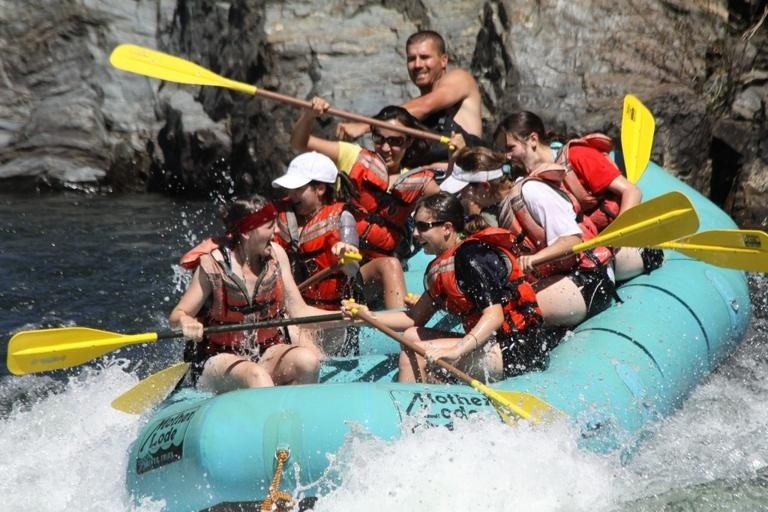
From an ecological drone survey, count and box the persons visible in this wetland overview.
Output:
[341,110,664,385]
[167,104,468,390]
[331,28,483,167]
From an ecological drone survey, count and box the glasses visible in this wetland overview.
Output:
[407,215,447,232]
[372,129,411,147]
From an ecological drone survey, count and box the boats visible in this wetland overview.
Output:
[116,126,752,511]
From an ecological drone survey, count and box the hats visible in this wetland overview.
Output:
[439,160,504,195]
[270,149,340,191]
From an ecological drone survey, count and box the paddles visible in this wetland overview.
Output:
[532,190,700,270]
[341,303,562,431]
[5,300,366,377]
[110,43,453,151]
[621,93,656,185]
[111,248,362,415]
[659,230,767,273]
